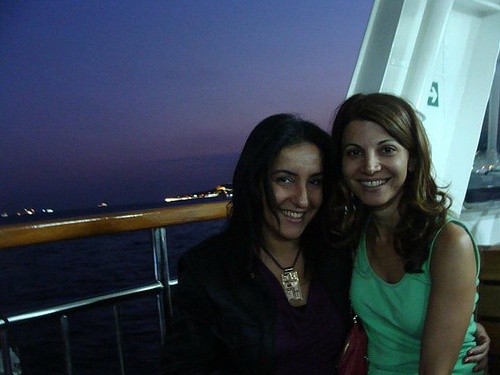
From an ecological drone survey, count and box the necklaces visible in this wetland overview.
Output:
[257,236,304,302]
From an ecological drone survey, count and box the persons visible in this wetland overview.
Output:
[160,113,491,375]
[324,92,484,374]
